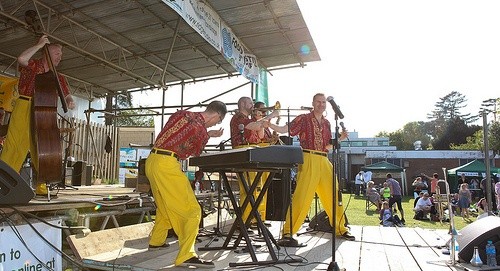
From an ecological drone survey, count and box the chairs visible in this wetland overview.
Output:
[364,188,382,212]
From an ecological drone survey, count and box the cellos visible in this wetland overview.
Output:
[24,9,62,202]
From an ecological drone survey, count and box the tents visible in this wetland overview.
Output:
[447,160,500,196]
[361,161,408,197]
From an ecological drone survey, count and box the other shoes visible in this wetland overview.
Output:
[400,218,405,223]
[235,226,253,234]
[178,256,215,268]
[284,236,298,245]
[253,220,271,226]
[148,242,170,251]
[250,225,260,230]
[341,231,356,241]
[35,192,57,197]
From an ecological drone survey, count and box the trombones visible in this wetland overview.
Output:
[231,101,327,118]
[436,168,457,266]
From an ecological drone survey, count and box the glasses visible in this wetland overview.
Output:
[215,109,222,124]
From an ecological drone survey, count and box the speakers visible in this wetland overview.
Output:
[309,209,348,233]
[87,166,93,186]
[447,215,500,265]
[0,158,36,206]
[266,136,293,221]
[72,161,87,186]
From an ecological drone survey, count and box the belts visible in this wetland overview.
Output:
[303,149,327,156]
[19,96,32,101]
[240,143,256,145]
[260,141,269,143]
[150,148,178,159]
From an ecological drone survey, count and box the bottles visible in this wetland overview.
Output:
[486,241,497,268]
[195,180,201,195]
[470,247,483,267]
[211,182,215,193]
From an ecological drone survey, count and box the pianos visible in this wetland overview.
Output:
[188,146,305,268]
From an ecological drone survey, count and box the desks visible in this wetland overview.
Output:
[197,161,304,267]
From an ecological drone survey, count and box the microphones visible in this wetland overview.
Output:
[238,123,244,142]
[327,95,344,119]
[340,122,349,140]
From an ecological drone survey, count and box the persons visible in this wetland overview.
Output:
[0,34,75,198]
[262,93,355,245]
[380,182,397,212]
[230,96,279,233]
[192,168,204,190]
[145,101,228,269]
[411,173,439,220]
[457,173,500,221]
[378,202,405,227]
[386,173,405,223]
[366,181,383,210]
[356,170,363,197]
[363,171,372,183]
